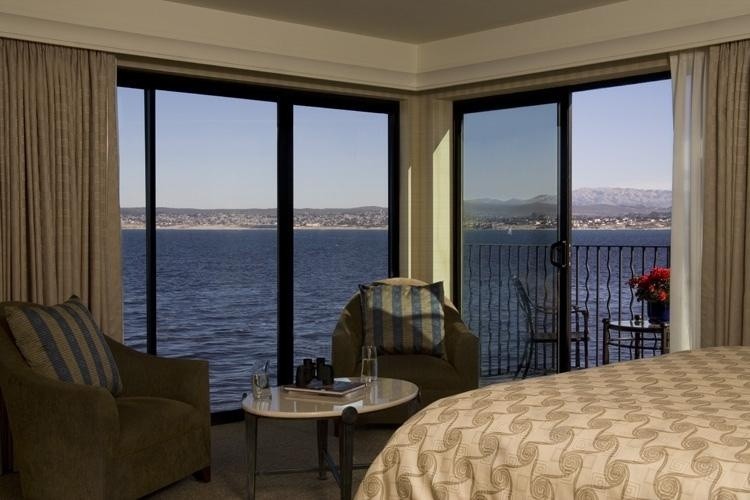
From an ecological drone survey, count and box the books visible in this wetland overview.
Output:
[284,380,365,395]
[285,391,367,407]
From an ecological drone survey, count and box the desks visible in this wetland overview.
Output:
[602,315,670,365]
[240,377,420,500]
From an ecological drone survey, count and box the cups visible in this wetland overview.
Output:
[359,347,378,381]
[251,373,272,401]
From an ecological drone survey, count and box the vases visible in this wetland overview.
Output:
[648,299,670,324]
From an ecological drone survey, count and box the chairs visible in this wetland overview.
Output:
[331,277,480,437]
[0,302,212,500]
[512,276,590,381]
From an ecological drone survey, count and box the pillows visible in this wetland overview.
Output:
[4,294,122,397]
[357,282,447,362]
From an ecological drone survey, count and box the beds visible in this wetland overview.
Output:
[352,346,750,500]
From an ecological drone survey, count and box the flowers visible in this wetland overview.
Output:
[625,267,670,301]
